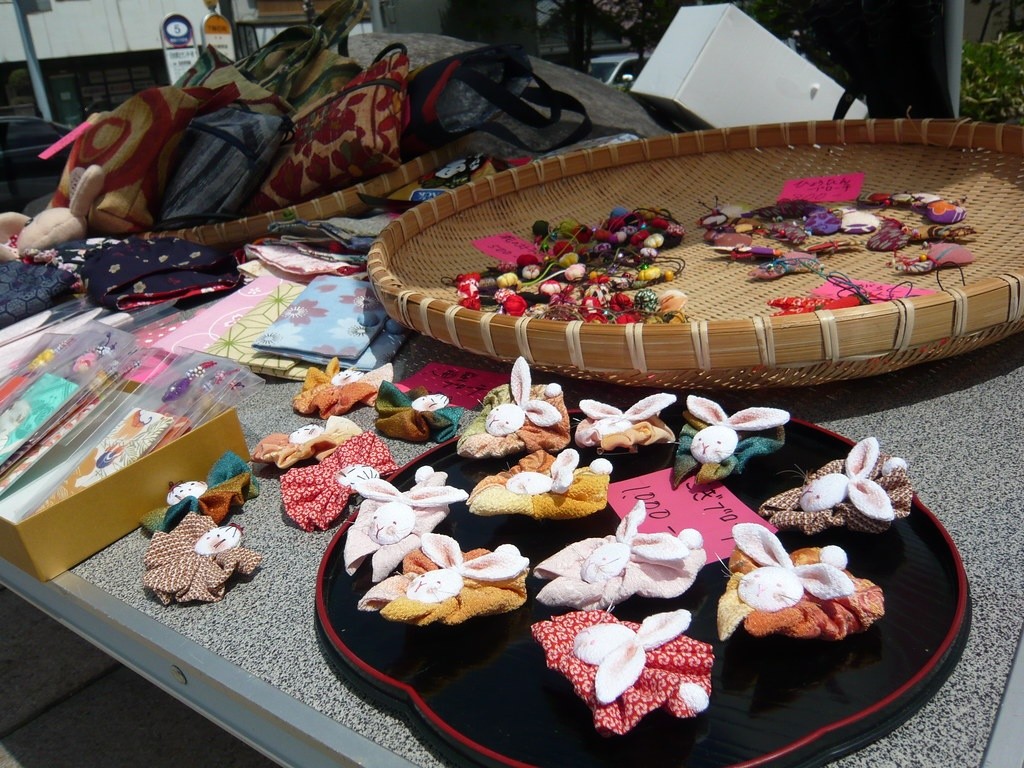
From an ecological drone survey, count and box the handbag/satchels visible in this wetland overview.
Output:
[154,99,295,231]
[46,81,241,234]
[241,0,369,112]
[240,42,408,215]
[172,24,327,116]
[400,42,593,152]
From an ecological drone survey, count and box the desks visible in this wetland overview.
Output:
[0,279,1024,768]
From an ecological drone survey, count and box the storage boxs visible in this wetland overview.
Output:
[629,3,870,134]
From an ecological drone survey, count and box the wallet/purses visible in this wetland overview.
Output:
[151,275,287,357]
[252,276,412,371]
[202,281,326,381]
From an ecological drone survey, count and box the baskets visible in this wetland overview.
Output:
[132,132,477,254]
[367,118,1024,390]
